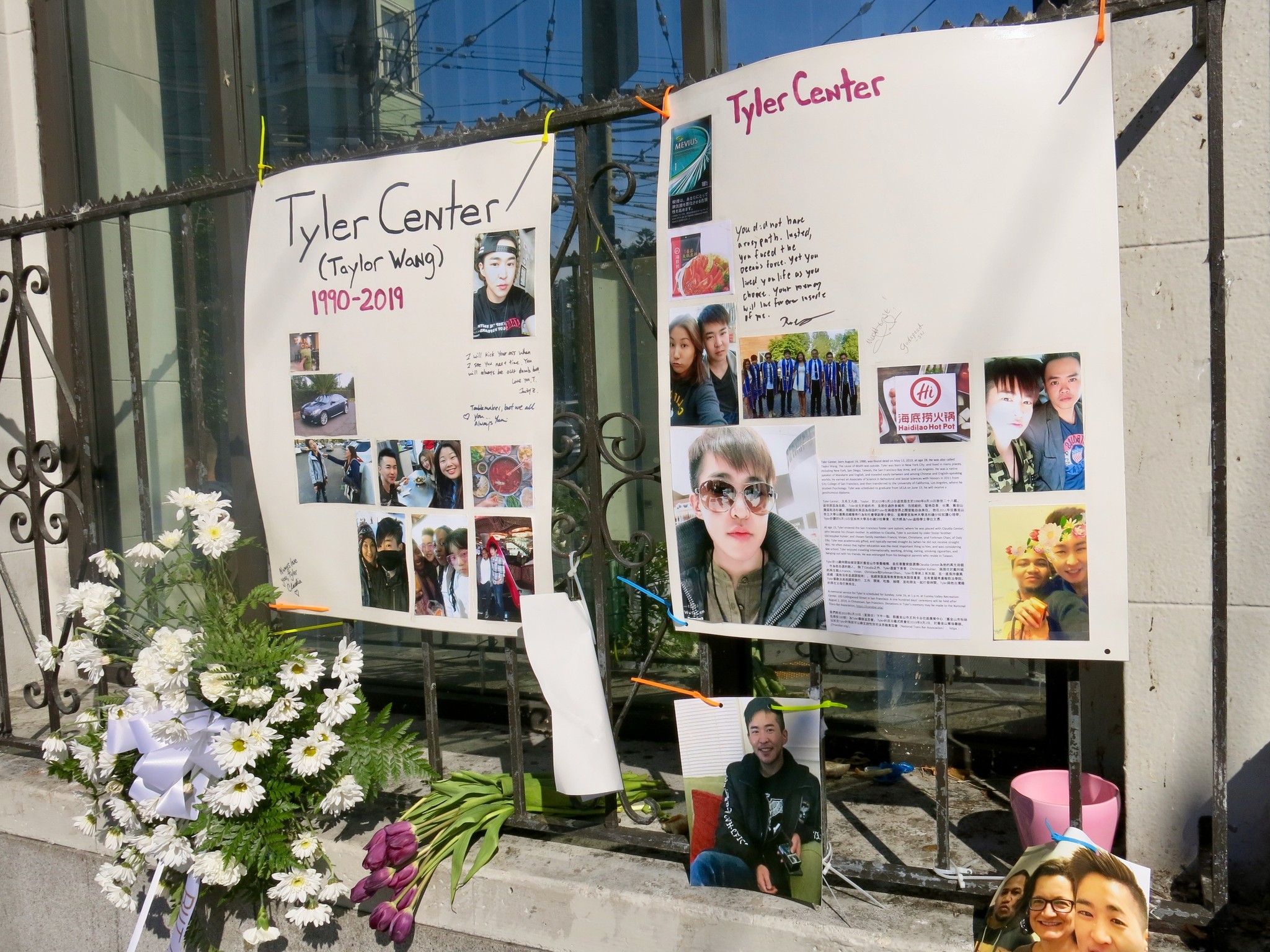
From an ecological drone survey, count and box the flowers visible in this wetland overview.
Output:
[34,485,442,943]
[348,772,676,942]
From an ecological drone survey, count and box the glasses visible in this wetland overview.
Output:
[446,550,468,567]
[694,480,779,517]
[419,544,433,550]
[766,356,772,359]
[1026,897,1074,914]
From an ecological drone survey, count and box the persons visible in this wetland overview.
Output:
[472,230,535,338]
[992,507,1088,640]
[691,697,820,896]
[741,348,860,418]
[305,439,328,502]
[419,440,464,510]
[476,495,499,508]
[378,448,410,507]
[323,445,362,502]
[521,458,532,470]
[476,542,506,618]
[973,845,1148,952]
[668,304,739,426]
[411,525,469,619]
[675,427,826,630]
[298,355,311,371]
[358,517,409,613]
[984,352,1084,492]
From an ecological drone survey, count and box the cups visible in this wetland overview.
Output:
[301,348,312,371]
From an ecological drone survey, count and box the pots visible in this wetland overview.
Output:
[488,454,523,497]
[939,363,971,442]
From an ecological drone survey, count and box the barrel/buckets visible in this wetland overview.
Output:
[1009,770,1121,856]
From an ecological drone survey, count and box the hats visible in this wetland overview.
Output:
[477,231,520,258]
[358,521,377,588]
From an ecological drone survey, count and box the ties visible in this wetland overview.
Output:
[814,361,818,379]
[786,361,789,386]
[828,365,832,385]
[844,364,847,383]
[769,365,773,383]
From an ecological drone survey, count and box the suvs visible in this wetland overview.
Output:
[343,439,372,473]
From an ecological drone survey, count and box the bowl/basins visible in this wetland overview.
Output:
[415,476,426,485]
[675,256,698,297]
[477,462,487,474]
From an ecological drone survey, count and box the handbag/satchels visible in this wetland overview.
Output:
[344,484,359,502]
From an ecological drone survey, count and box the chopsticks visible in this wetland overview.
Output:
[507,457,531,475]
[494,485,502,507]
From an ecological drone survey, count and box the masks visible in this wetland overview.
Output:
[375,550,401,570]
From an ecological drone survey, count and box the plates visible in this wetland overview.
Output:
[473,474,490,498]
[517,445,532,465]
[470,446,485,461]
[486,491,505,507]
[883,376,920,443]
[486,446,511,456]
[918,364,948,375]
[519,487,533,508]
[505,495,523,507]
[878,401,890,437]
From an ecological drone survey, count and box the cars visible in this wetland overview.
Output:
[295,442,324,454]
[300,394,349,426]
[325,442,342,452]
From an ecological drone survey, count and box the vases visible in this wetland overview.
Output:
[1010,770,1121,859]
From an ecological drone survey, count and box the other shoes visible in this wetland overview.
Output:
[759,415,766,418]
[810,413,815,417]
[801,409,806,417]
[485,611,489,619]
[817,413,822,416]
[769,410,776,418]
[753,413,758,418]
[478,610,483,614]
[787,411,793,415]
[779,414,785,418]
